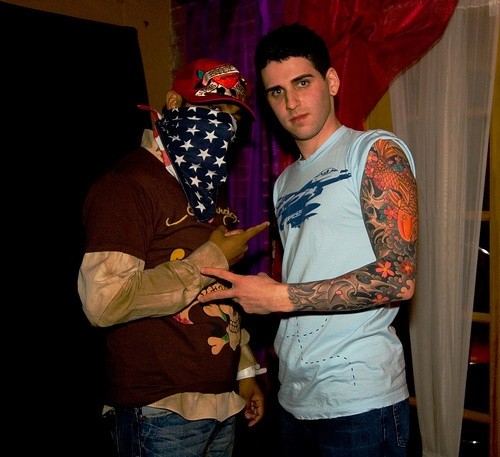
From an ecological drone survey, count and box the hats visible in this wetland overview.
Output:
[170,58,259,122]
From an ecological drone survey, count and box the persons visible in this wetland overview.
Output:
[196,21,422,456]
[74,59,275,456]
